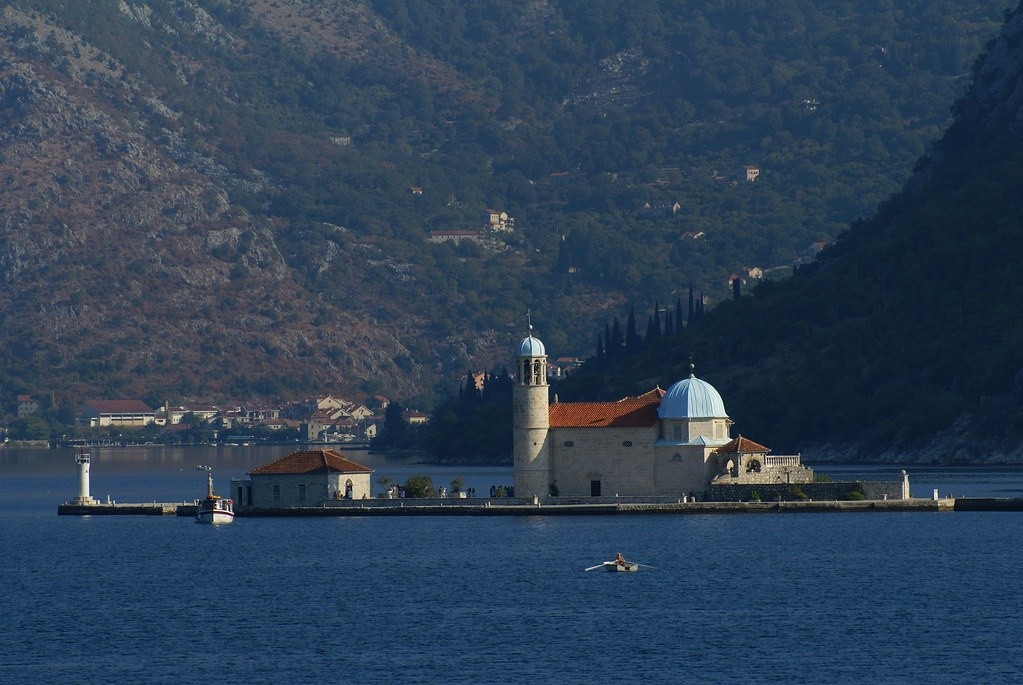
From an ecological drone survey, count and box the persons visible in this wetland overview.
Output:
[362,494,366,499]
[615,553,624,566]
[552,480,557,488]
[472,488,475,497]
[439,485,443,497]
[400,488,405,498]
[490,484,514,497]
[333,490,352,499]
[387,483,399,498]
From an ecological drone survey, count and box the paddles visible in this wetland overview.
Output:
[585,560,619,571]
[622,560,654,568]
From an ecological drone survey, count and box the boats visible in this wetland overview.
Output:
[198,495,234,524]
[197,465,212,471]
[603,562,638,572]
